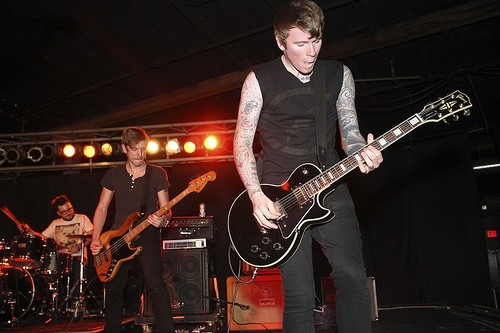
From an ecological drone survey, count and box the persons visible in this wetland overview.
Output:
[17,195,93,322]
[90,127,174,333]
[232,0,384,333]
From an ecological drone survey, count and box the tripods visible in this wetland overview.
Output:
[42,238,105,328]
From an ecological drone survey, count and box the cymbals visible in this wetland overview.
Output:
[65,233,92,238]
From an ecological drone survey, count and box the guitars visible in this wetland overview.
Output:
[227,89,472,269]
[94,169,217,282]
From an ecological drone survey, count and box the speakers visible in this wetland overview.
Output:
[143,248,210,316]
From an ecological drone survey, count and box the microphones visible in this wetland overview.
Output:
[67,234,92,238]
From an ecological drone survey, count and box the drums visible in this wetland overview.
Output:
[0,263,35,327]
[9,256,40,277]
[66,295,96,312]
[10,235,35,249]
[41,253,68,276]
